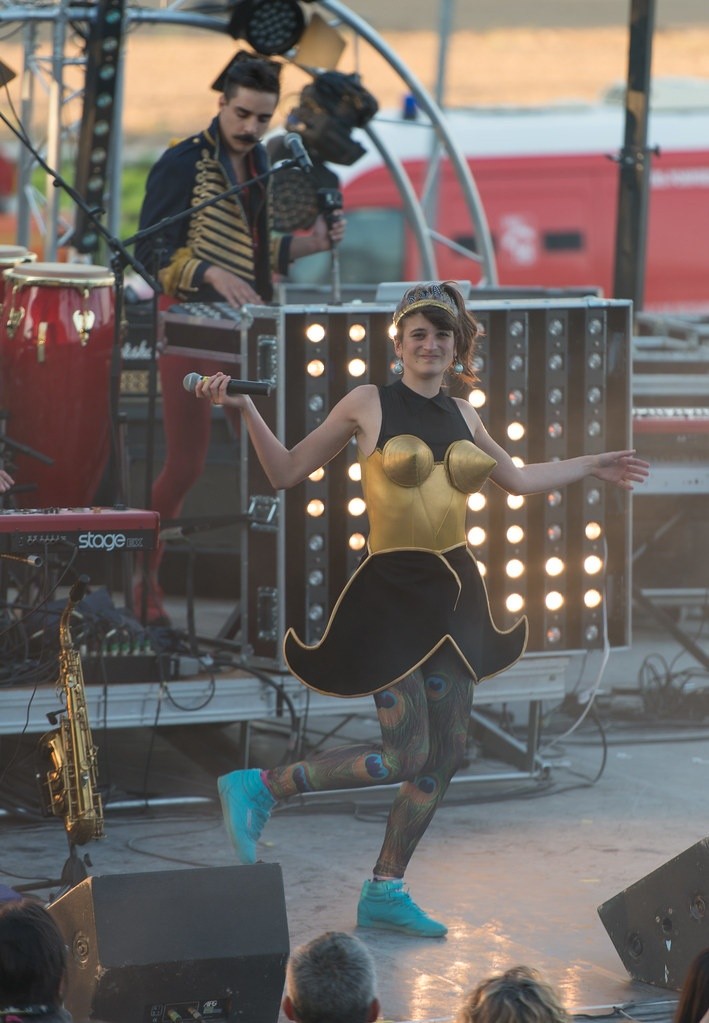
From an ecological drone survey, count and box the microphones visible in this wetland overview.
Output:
[284,132,315,174]
[183,372,272,397]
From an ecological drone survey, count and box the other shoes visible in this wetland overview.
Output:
[132,581,165,624]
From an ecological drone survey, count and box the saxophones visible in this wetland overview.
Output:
[38,574,110,847]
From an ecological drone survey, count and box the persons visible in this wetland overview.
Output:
[0,469,14,494]
[127,53,345,628]
[0,883,709,1023]
[192,281,651,938]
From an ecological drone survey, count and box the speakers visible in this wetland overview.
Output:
[46,861,290,1023]
[597,835,709,992]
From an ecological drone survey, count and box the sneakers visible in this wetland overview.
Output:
[355,881,448,938]
[217,767,278,864]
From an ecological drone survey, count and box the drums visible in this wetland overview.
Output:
[0,243,41,264]
[0,261,118,509]
